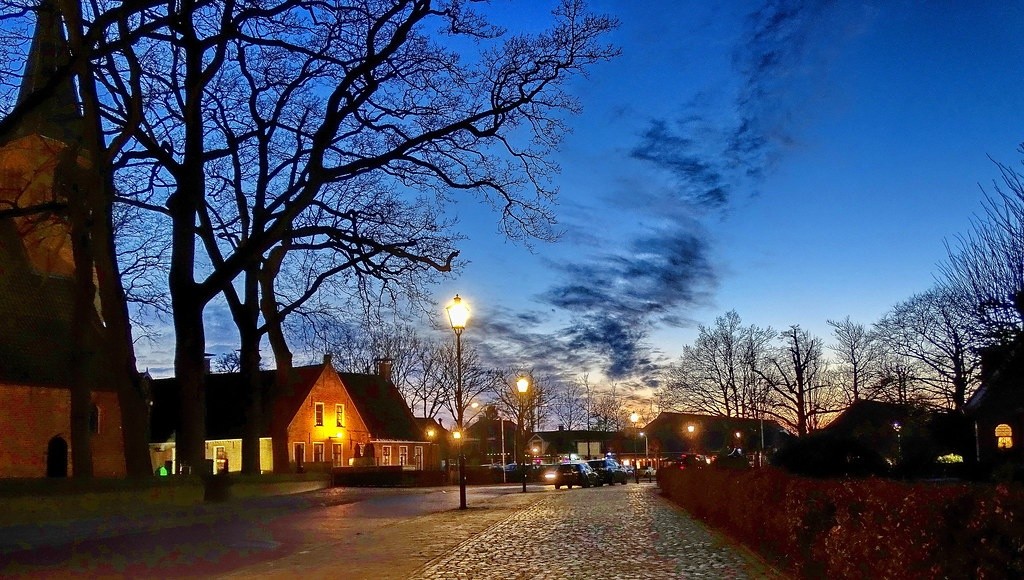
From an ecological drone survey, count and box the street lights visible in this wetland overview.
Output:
[444,292,472,511]
[640,432,649,465]
[514,375,531,491]
[630,411,639,484]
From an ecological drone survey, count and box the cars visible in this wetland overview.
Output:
[588,458,628,485]
[681,452,707,470]
[553,461,600,490]
